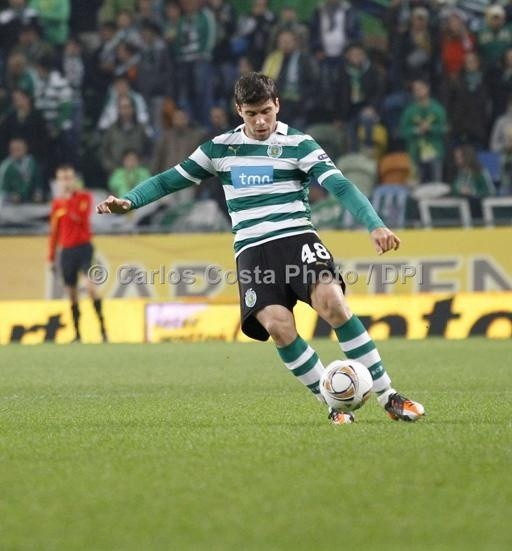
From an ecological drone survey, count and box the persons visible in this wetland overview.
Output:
[44,155,111,345]
[0,0,511,232]
[95,72,426,425]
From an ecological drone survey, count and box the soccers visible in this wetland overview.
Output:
[320,359,373,412]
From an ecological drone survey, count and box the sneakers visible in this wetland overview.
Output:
[377,389,425,421]
[328,406,354,425]
